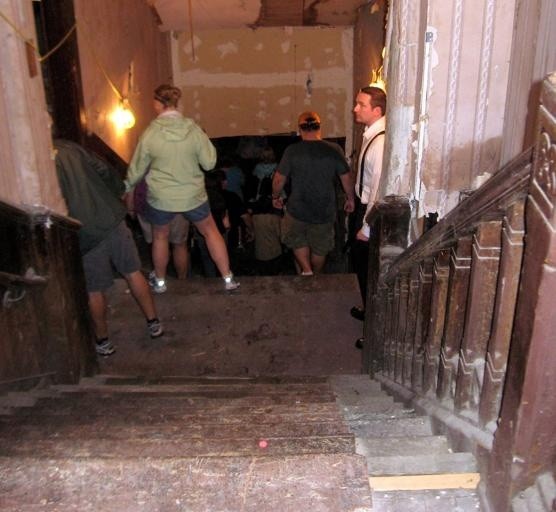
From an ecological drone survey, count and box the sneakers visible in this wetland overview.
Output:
[94,339,116,355]
[147,275,167,295]
[356,338,365,349]
[223,273,241,290]
[147,322,164,338]
[350,305,365,321]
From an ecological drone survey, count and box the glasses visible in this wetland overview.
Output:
[300,122,320,130]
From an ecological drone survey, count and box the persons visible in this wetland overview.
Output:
[125,85,242,293]
[52,139,162,355]
[350,86,387,348]
[238,111,354,276]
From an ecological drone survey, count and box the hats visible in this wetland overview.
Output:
[298,111,321,127]
